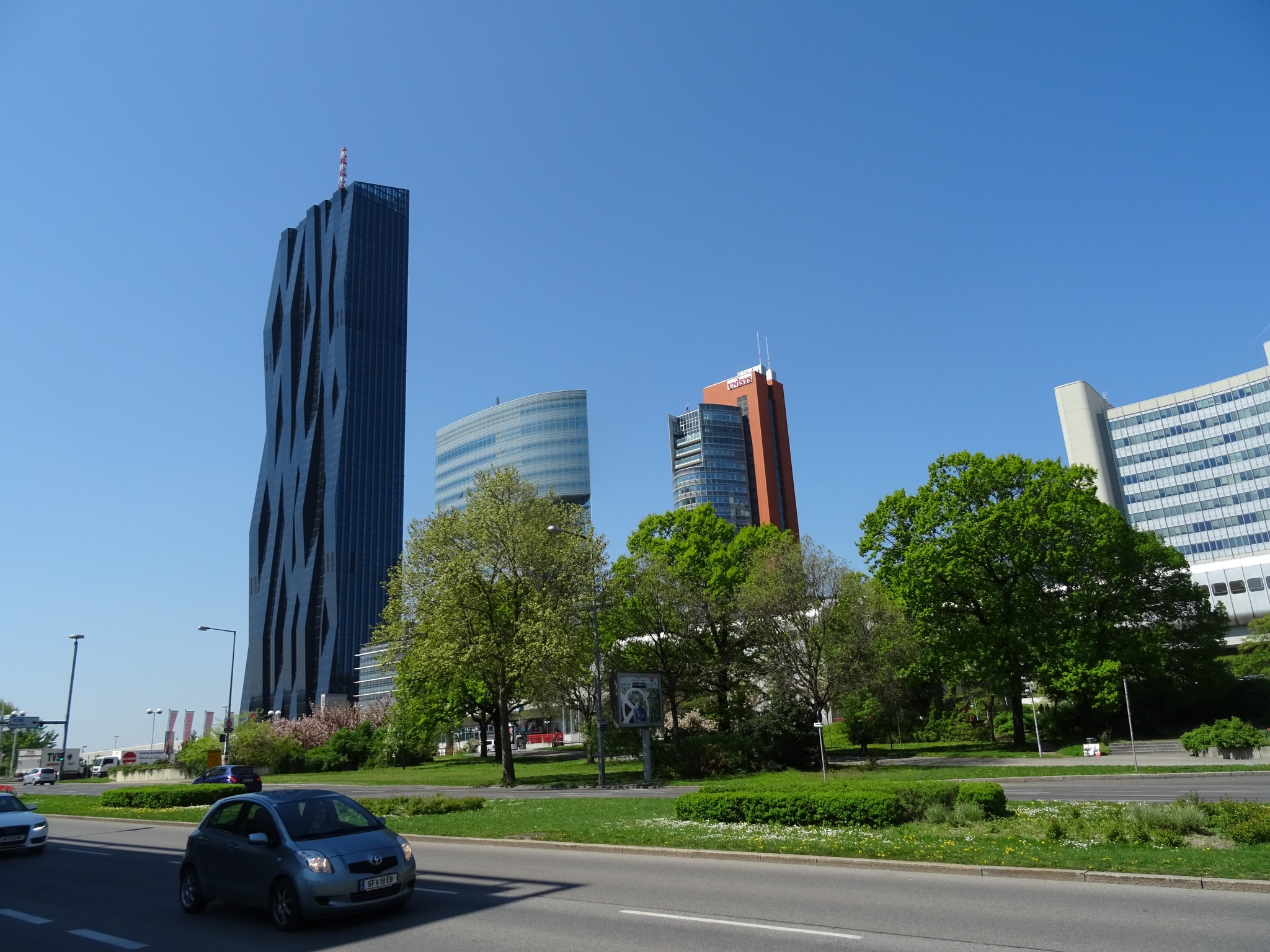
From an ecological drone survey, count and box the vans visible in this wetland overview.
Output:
[476,739,496,753]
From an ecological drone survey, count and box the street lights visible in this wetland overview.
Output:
[544,720,551,736]
[9,710,27,776]
[146,708,163,750]
[114,735,119,749]
[547,525,607,786]
[82,745,87,753]
[198,625,237,766]
[1024,688,1043,758]
[57,634,85,781]
[220,704,232,712]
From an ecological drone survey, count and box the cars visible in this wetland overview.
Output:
[178,787,418,932]
[191,764,262,794]
[22,766,57,786]
[0,792,49,857]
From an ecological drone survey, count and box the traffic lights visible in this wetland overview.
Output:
[59,751,65,761]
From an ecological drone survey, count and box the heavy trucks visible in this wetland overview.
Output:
[14,748,85,781]
[92,749,176,778]
